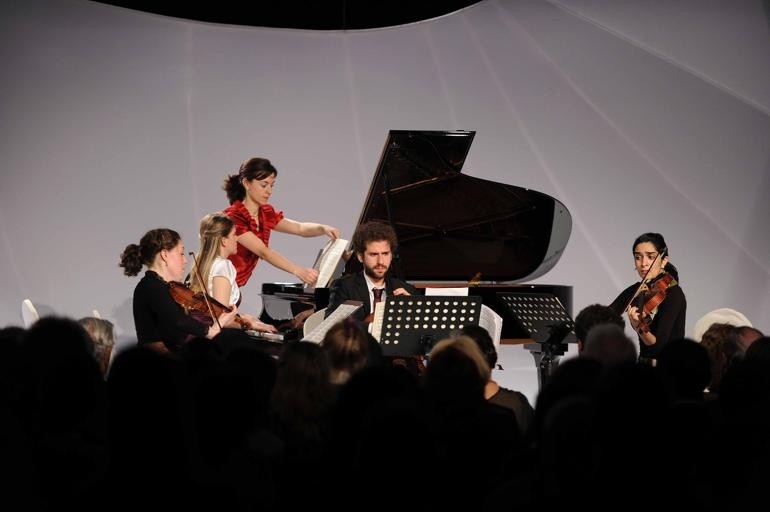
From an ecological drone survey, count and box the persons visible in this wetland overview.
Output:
[585,324,637,365]
[119,228,277,358]
[455,326,532,425]
[575,303,625,358]
[380,334,521,512]
[0,318,249,512]
[325,221,421,342]
[603,233,684,364]
[215,157,339,284]
[246,330,376,512]
[521,356,707,511]
[190,215,276,332]
[655,324,770,512]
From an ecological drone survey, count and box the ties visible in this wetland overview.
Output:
[372,288,385,310]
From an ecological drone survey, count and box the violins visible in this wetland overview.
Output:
[167,280,253,338]
[630,272,674,334]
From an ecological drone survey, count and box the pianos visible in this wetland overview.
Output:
[260,129,574,344]
